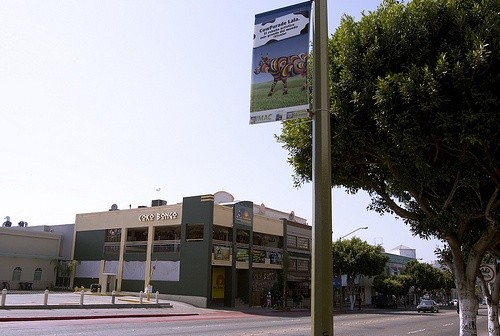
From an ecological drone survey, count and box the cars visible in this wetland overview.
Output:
[437,298,458,310]
[481,299,486,305]
[416,299,440,313]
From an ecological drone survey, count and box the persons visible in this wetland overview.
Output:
[266,292,272,309]
[293,293,304,308]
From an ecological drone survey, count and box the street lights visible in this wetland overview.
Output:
[339,227,368,310]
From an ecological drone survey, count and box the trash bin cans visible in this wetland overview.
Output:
[19,282,33,290]
